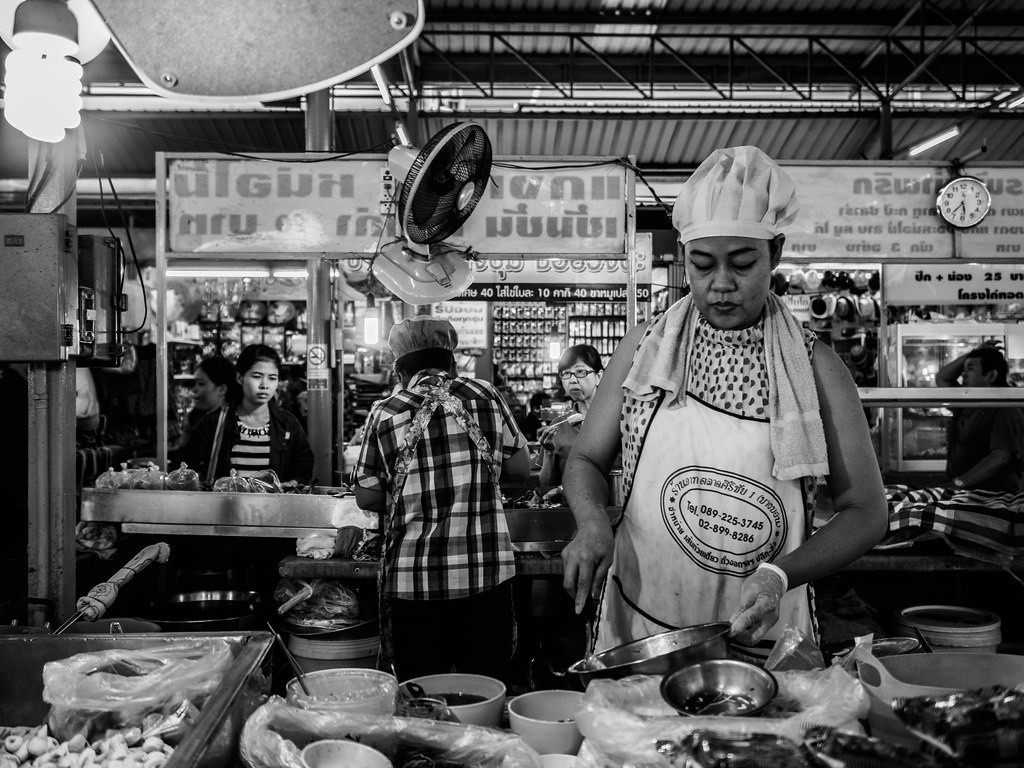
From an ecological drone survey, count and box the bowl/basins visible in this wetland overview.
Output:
[133,589,263,630]
[397,673,506,728]
[831,636,921,661]
[660,658,778,718]
[302,740,393,768]
[287,667,396,717]
[508,690,585,756]
[566,619,731,688]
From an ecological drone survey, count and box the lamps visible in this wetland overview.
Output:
[908,125,961,157]
[370,65,492,245]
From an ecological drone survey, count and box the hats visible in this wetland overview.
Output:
[388,316,459,360]
[670,146,799,241]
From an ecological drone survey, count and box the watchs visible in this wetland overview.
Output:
[954,478,965,488]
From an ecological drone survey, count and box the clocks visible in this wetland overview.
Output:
[936,176,992,229]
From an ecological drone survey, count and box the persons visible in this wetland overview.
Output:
[283,363,309,429]
[132,343,183,454]
[352,315,533,678]
[187,344,315,486]
[848,338,883,458]
[168,359,238,460]
[561,145,889,665]
[526,386,552,440]
[537,345,624,493]
[936,340,1020,492]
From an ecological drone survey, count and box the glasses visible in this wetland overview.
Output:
[559,370,598,380]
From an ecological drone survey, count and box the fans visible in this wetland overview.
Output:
[372,122,492,305]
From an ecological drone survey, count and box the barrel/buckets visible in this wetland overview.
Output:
[289,635,381,677]
[896,604,1002,656]
